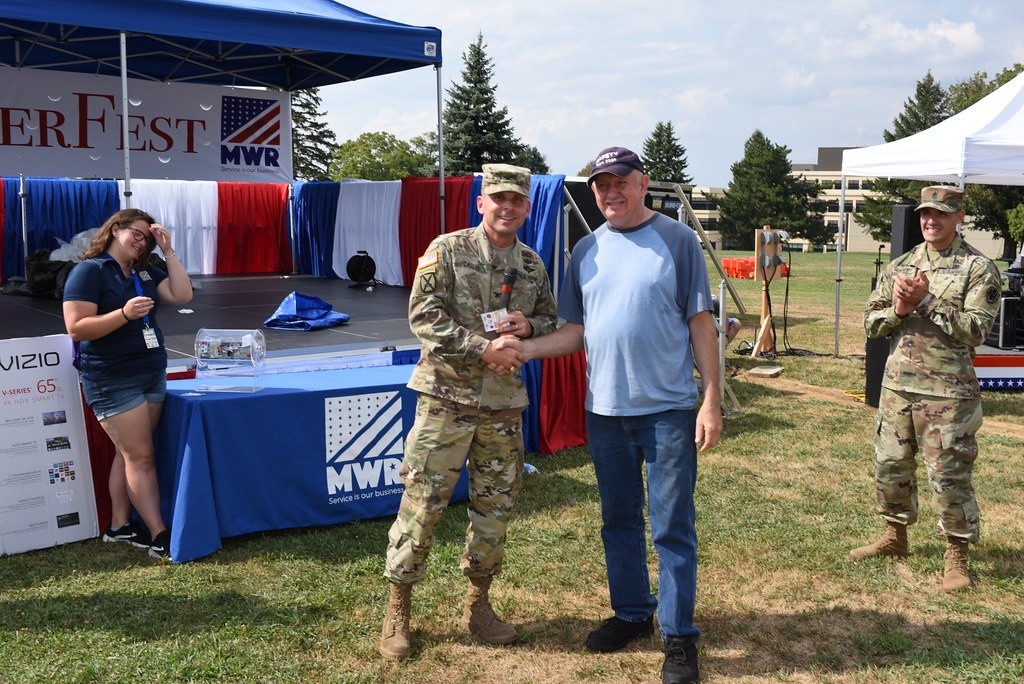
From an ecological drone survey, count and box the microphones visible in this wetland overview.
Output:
[499,266,518,311]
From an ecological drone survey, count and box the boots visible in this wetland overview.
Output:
[461,575,519,645]
[850,522,909,561]
[942,537,971,594]
[380,582,412,660]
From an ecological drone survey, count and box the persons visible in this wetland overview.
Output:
[480,148,724,684]
[711,294,742,377]
[380,164,558,663]
[849,186,1001,592]
[63,209,193,561]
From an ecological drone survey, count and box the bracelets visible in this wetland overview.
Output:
[122,307,131,322]
[162,248,176,257]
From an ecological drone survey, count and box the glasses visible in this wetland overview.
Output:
[123,227,153,245]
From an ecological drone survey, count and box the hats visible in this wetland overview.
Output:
[586,146,644,188]
[913,185,965,212]
[482,164,530,198]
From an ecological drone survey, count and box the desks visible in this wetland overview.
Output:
[131,364,473,565]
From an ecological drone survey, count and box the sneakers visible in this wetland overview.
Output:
[660,635,699,684]
[148,529,171,561]
[102,518,151,548]
[586,616,655,652]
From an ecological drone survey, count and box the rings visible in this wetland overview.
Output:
[515,324,519,330]
[508,366,515,373]
[899,289,903,295]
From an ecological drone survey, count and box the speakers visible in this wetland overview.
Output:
[866,336,890,408]
[889,203,927,263]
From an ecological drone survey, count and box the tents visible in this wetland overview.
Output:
[0,0,445,236]
[833,70,1024,358]
